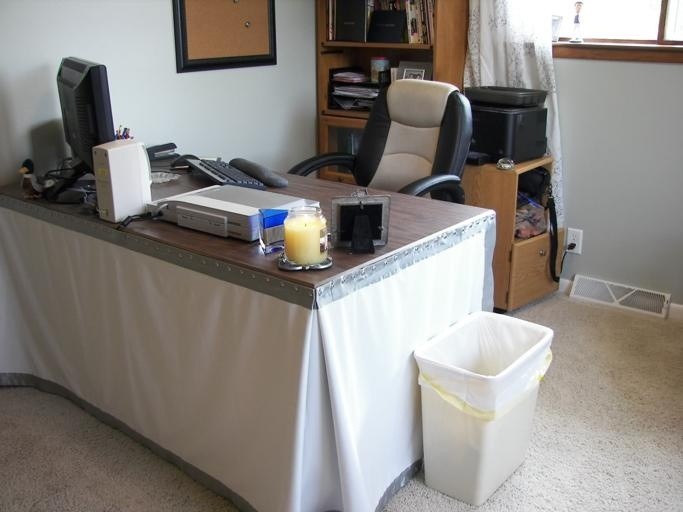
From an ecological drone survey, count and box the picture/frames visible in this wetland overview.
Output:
[170,1,277,74]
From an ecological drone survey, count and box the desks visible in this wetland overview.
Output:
[0,161,495,511]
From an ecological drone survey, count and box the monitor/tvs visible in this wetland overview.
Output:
[46,57,115,205]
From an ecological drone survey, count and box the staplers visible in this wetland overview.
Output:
[146,143,180,162]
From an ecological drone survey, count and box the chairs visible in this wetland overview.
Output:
[287,78,472,204]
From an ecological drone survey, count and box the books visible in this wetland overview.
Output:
[372,0,435,45]
[331,70,379,111]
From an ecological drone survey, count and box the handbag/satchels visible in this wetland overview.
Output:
[518,167,559,284]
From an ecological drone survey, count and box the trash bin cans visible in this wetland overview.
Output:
[414,310,553,507]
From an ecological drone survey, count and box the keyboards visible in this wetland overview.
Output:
[184,158,268,191]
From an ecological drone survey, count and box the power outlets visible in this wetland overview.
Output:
[565,228,582,255]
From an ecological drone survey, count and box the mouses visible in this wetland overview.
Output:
[171,154,199,167]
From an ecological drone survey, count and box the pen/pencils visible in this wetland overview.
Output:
[114,124,130,141]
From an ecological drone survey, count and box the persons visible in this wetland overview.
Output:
[414,74,422,80]
[406,73,415,79]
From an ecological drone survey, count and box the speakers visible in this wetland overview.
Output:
[91,139,151,222]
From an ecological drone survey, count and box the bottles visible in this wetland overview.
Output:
[370,56,390,82]
[283,206,328,263]
